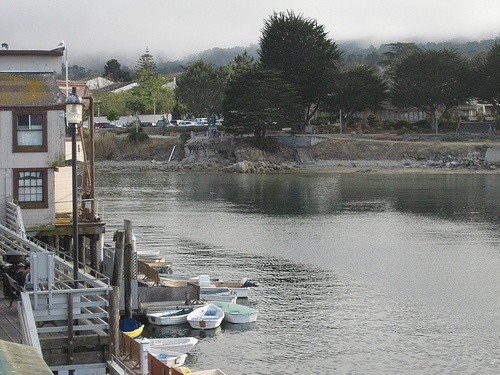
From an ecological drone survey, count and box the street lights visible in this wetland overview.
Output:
[64,85,85,336]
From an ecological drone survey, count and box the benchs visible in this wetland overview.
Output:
[193,315,219,319]
[4,273,29,307]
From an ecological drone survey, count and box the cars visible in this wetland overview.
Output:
[94,122,122,129]
[140,117,225,128]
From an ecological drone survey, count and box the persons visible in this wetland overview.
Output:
[0,255,7,268]
[15,262,30,295]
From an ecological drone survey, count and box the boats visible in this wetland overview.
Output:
[131,336,228,375]
[118,249,259,338]
